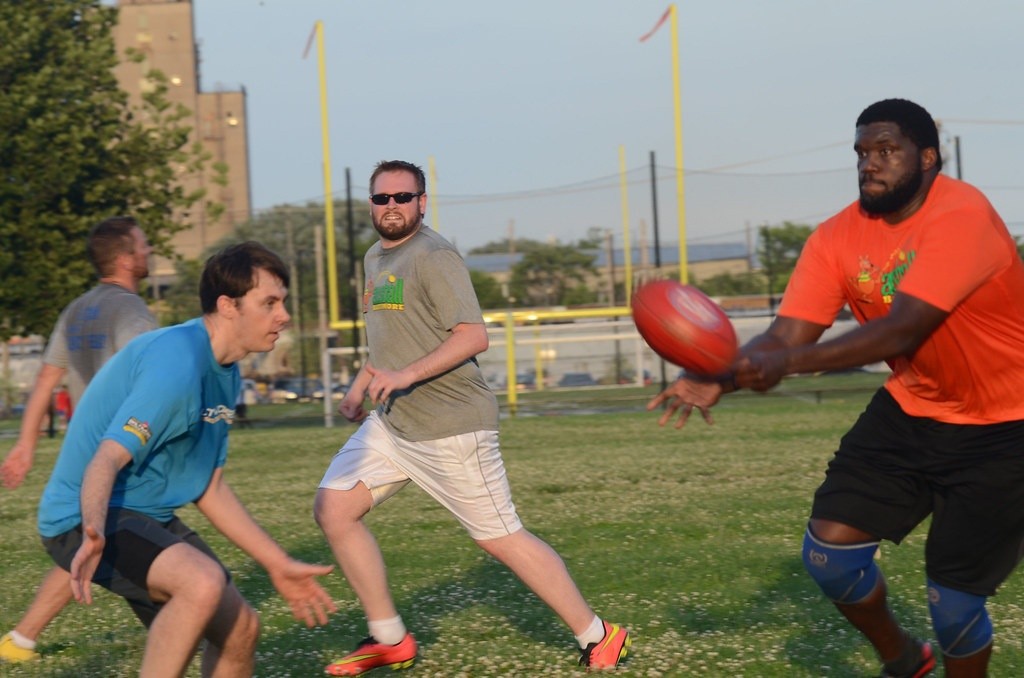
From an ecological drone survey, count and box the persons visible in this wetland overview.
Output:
[646,99,1024,678]
[0,216,336,678]
[315,160,632,678]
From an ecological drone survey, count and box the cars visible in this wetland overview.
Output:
[233,377,351,407]
[499,368,656,390]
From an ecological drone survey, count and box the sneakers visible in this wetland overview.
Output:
[578,620,632,675]
[880,636,938,678]
[0,633,41,665]
[324,632,418,678]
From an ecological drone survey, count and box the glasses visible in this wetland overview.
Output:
[369,191,422,205]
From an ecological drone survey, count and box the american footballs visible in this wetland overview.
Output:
[631,280,735,378]
